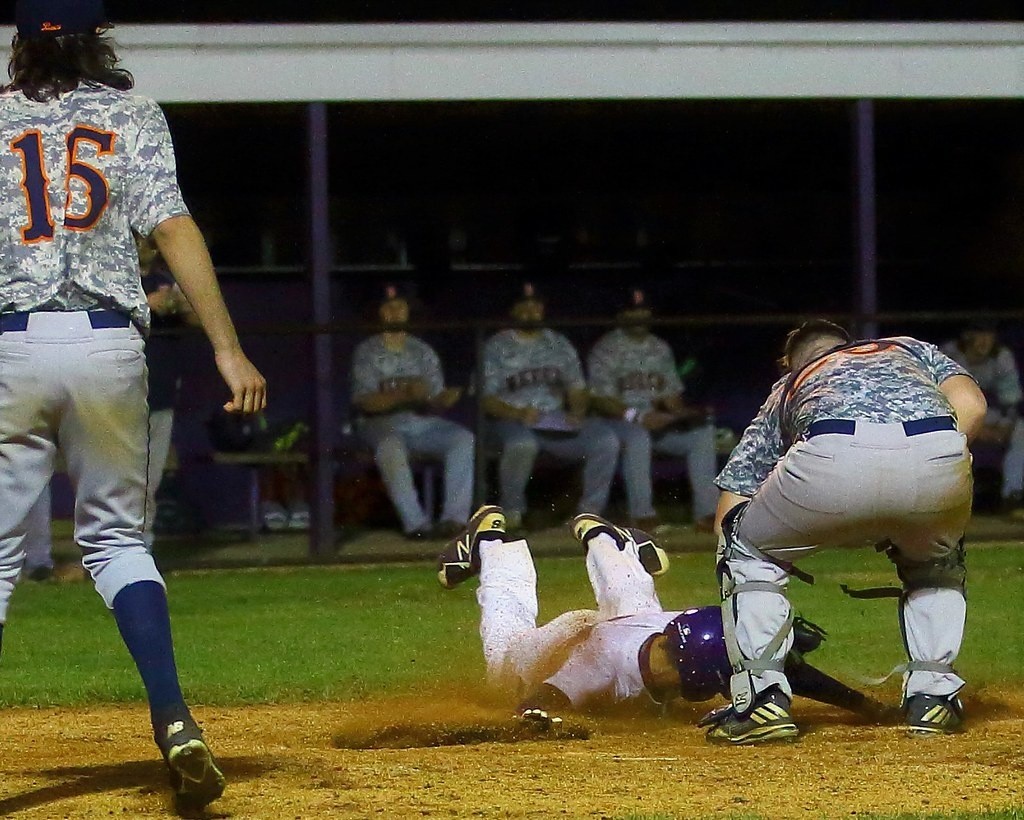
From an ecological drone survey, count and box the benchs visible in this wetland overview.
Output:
[202,447,442,530]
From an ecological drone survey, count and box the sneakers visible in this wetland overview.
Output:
[697,691,800,745]
[570,511,670,575]
[153,710,226,813]
[904,695,965,737]
[435,503,508,593]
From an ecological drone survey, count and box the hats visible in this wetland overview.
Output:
[508,281,550,307]
[610,284,651,310]
[376,283,413,306]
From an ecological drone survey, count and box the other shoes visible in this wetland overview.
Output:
[630,516,655,533]
[407,522,464,543]
[262,503,311,529]
[693,513,715,537]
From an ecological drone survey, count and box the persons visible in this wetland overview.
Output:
[0,21,270,812]
[438,504,905,745]
[931,318,1024,529]
[351,280,718,539]
[705,317,991,746]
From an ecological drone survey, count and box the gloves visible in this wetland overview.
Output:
[883,705,906,726]
[515,681,570,716]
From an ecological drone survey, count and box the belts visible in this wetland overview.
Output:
[0,309,136,332]
[807,418,960,436]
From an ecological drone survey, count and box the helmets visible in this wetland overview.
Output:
[658,605,730,704]
